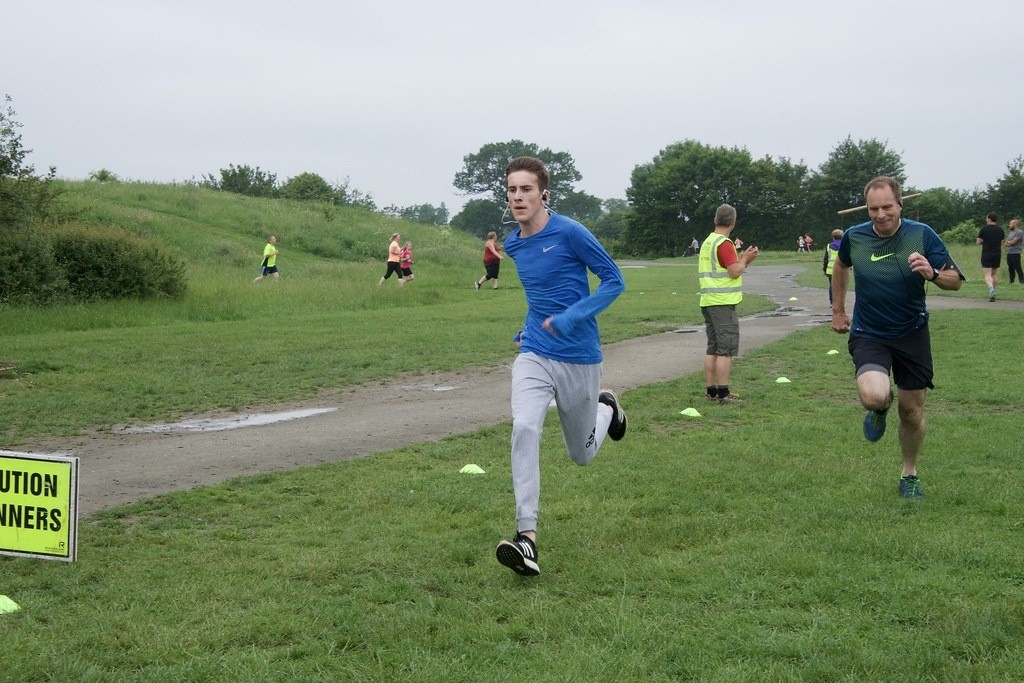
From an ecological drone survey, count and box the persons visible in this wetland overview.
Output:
[976,211,1004,302]
[1004,219,1024,286]
[698,204,759,405]
[796,235,807,254]
[474,229,502,290]
[822,229,845,308]
[734,237,744,253]
[804,233,814,253]
[496,156,628,577]
[377,233,402,284]
[399,241,416,283]
[253,234,279,283]
[689,237,700,256]
[831,175,968,498]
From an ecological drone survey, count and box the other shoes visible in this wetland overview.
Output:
[475,281,481,292]
[989,291,997,302]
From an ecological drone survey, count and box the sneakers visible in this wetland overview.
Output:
[899,468,923,499]
[599,388,627,441]
[863,390,894,442]
[496,530,540,577]
[704,393,722,402]
[716,394,748,405]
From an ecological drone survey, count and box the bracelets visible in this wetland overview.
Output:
[406,258,408,260]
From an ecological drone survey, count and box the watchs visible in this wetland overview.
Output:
[927,268,938,282]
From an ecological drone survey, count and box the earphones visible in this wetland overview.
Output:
[542,194,547,201]
[506,197,510,202]
[898,201,902,207]
